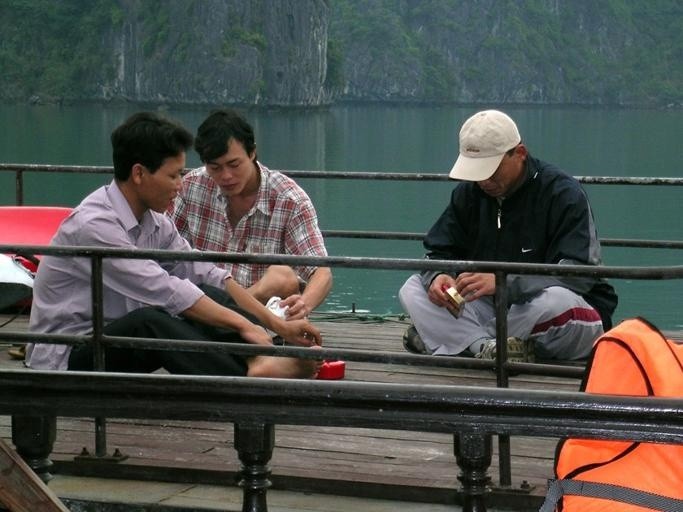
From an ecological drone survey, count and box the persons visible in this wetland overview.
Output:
[163,108,334,322]
[396,109,619,378]
[24,110,325,380]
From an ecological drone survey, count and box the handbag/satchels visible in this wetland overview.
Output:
[539,317,683,512]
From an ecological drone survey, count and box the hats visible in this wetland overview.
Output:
[449,110,521,182]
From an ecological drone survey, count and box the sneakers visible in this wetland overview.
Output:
[403,326,426,354]
[480,336,536,363]
